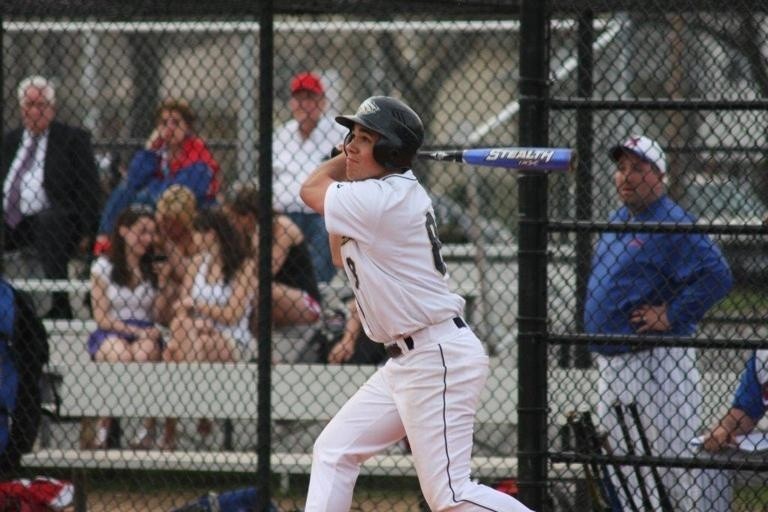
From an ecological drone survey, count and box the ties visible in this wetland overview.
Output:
[6,136,40,230]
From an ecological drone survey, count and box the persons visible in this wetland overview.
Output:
[0,277,51,482]
[305,95,540,512]
[0,74,107,321]
[582,133,732,512]
[273,74,349,282]
[689,346,768,512]
[96,99,223,235]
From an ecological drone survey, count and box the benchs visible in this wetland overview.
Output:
[5,236,768,512]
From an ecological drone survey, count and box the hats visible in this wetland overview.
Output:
[291,76,324,96]
[608,135,670,185]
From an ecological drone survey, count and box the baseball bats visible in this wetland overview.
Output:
[417,146,579,172]
[567,402,670,512]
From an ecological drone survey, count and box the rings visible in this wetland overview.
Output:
[642,316,646,321]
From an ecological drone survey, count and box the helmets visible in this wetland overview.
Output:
[335,95,424,172]
